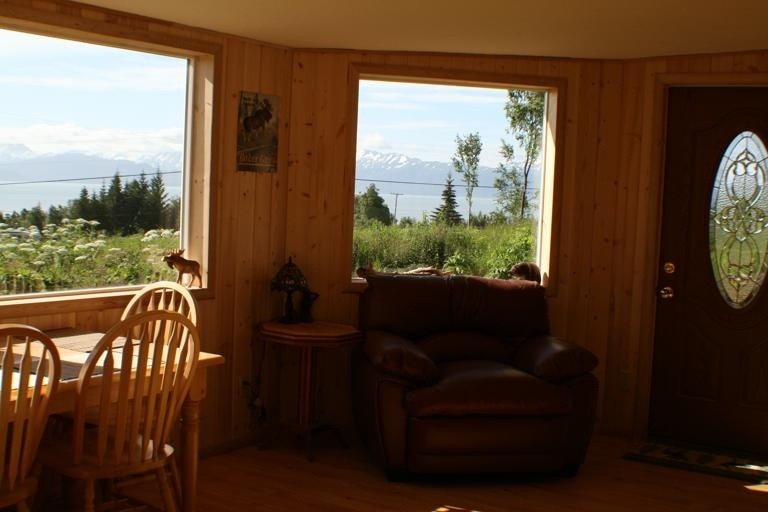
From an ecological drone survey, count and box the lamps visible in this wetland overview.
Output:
[270,257,308,325]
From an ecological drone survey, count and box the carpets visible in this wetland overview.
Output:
[627,444,768,483]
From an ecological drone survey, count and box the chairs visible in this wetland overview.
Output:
[120,281,197,348]
[1,323,63,511]
[46,308,201,512]
[354,266,600,476]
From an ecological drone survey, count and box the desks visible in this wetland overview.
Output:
[0,327,224,512]
[256,319,362,455]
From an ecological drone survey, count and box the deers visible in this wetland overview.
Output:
[164,253,202,289]
[243,100,274,142]
